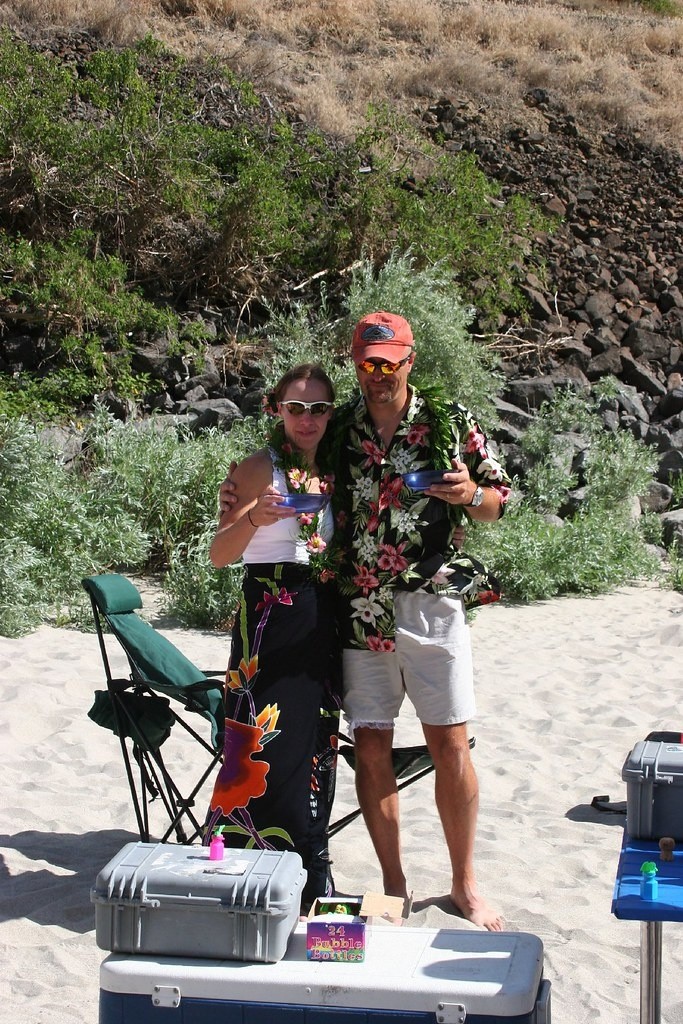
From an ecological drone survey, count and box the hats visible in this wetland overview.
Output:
[352,310,414,365]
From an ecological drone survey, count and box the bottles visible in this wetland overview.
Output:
[640,861,658,899]
[210,835,225,860]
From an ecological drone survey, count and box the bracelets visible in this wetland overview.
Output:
[247,509,259,528]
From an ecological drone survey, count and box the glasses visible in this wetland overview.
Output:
[357,352,411,375]
[279,400,333,417]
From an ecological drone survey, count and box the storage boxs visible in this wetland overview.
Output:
[89,842,306,964]
[308,890,424,962]
[98,922,551,1024]
[622,741,683,841]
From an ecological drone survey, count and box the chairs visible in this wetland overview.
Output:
[80,574,475,911]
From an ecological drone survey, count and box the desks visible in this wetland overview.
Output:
[611,826,683,1024]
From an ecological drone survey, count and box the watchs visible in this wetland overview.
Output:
[463,482,484,507]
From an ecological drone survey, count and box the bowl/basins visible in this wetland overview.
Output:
[401,470,460,489]
[275,493,331,514]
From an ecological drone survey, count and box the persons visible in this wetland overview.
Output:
[214,312,511,931]
[204,366,342,918]
